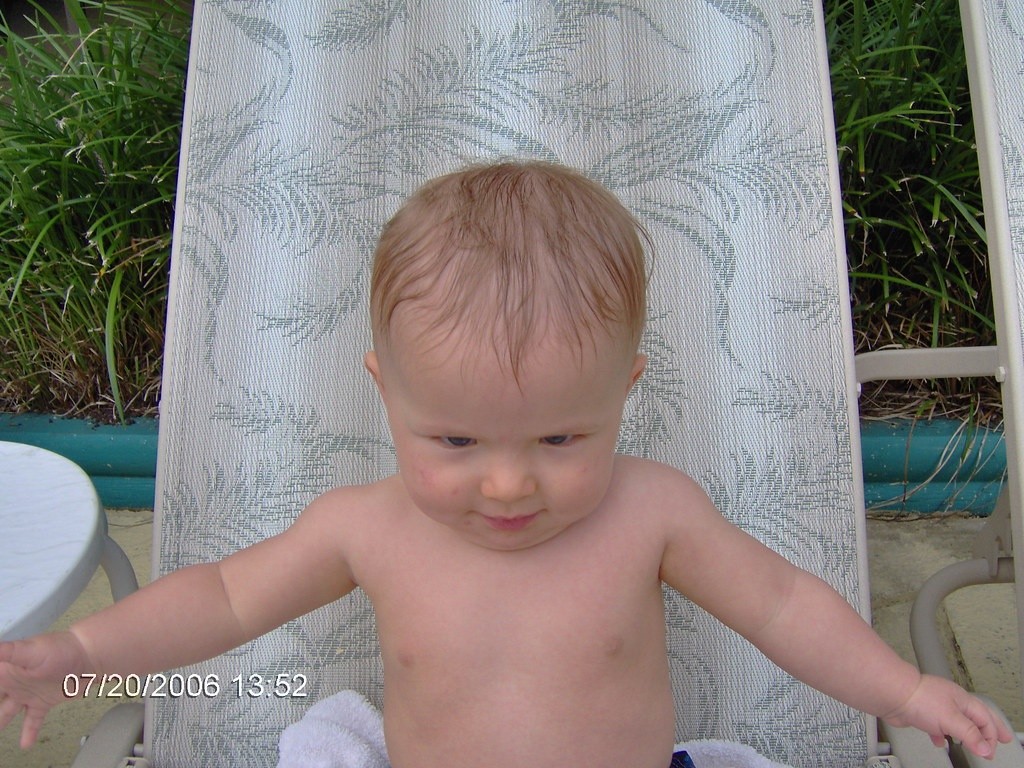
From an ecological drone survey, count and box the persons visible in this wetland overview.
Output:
[3,160,1014,768]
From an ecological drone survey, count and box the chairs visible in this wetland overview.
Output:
[856,0,1024,768]
[69,0,956,768]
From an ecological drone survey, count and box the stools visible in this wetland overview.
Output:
[0,439,139,641]
